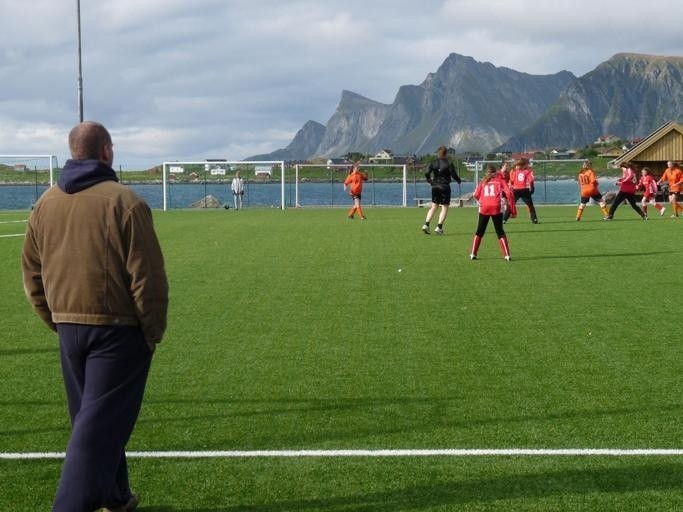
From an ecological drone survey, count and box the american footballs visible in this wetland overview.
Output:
[605,192,616,204]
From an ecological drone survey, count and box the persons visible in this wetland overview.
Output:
[231,170,245,210]
[602,161,649,220]
[469,156,539,261]
[422,145,461,236]
[634,167,666,216]
[343,162,368,220]
[575,159,609,221]
[656,160,683,217]
[21,120,170,512]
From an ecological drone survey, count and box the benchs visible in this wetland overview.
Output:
[412,196,471,208]
[614,189,672,204]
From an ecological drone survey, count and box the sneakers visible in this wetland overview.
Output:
[603,216,612,220]
[434,227,443,235]
[660,207,665,216]
[422,225,430,234]
